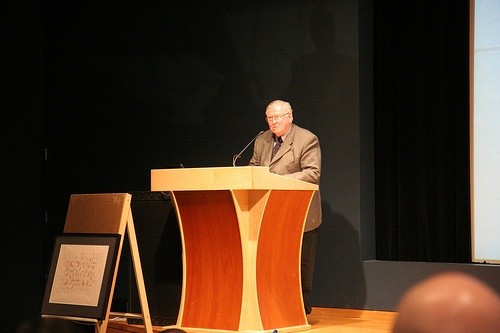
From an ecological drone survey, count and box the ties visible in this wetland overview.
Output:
[270,137,284,161]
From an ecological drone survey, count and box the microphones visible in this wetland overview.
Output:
[233,131,264,167]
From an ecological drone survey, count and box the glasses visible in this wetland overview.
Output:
[265,112,289,121]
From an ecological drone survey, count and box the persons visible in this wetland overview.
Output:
[388,268,500,333]
[248,99,324,313]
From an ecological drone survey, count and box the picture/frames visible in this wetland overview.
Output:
[38,232,122,319]
